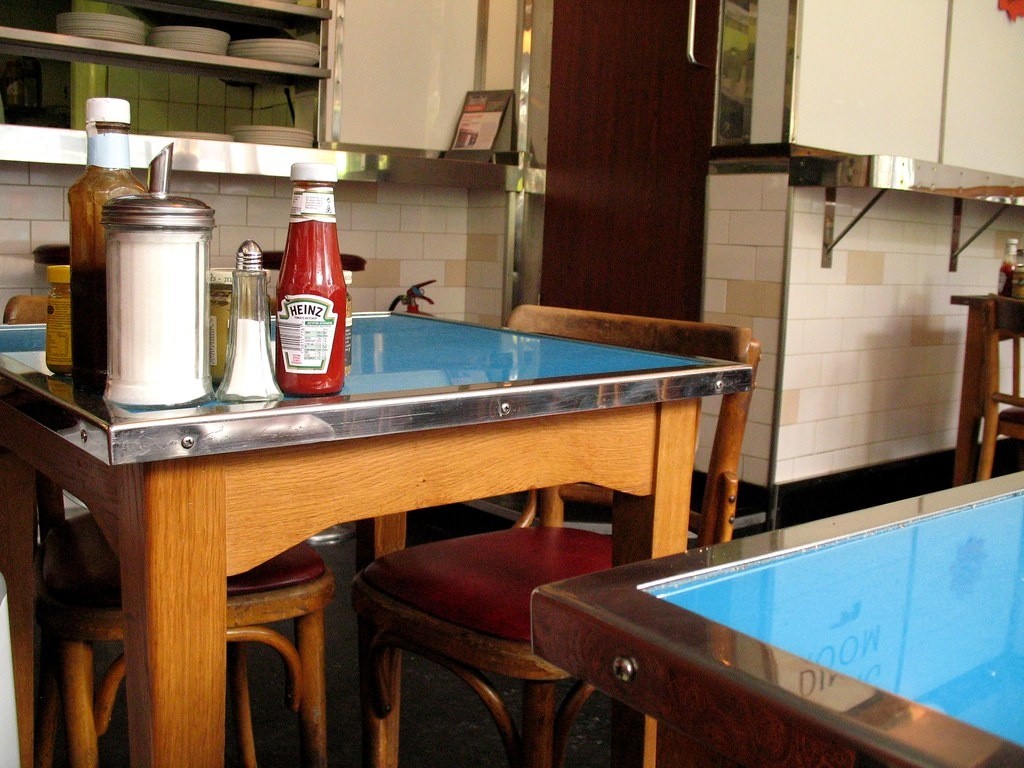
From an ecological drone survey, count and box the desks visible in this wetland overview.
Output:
[528,474,1024,768]
[948,292,1024,487]
[0,314,752,768]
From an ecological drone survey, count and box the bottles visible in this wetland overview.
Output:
[274,162,347,396]
[215,239,285,401]
[1011,248,1024,299]
[998,238,1018,297]
[101,143,214,407]
[68,96,148,408]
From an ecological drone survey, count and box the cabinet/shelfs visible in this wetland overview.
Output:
[0,0,335,146]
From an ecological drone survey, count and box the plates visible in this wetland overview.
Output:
[153,125,315,148]
[54,12,321,66]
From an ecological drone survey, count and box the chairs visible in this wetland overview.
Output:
[4,294,338,768]
[361,305,763,768]
[973,299,1024,486]
